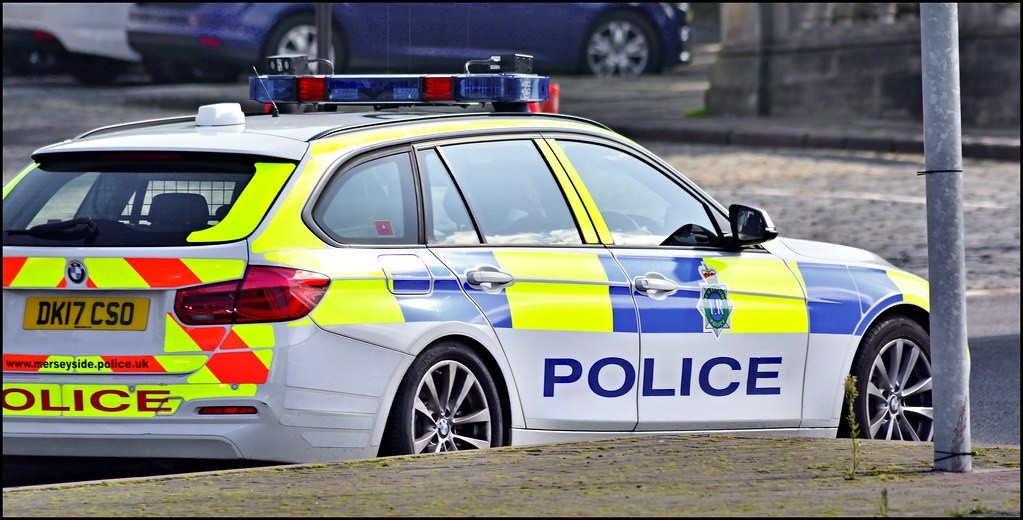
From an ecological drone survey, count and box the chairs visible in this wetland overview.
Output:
[443,172,513,235]
[146,193,210,233]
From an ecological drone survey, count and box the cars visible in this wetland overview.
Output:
[124,1,694,85]
[3,3,141,76]
[0,54,934,464]
